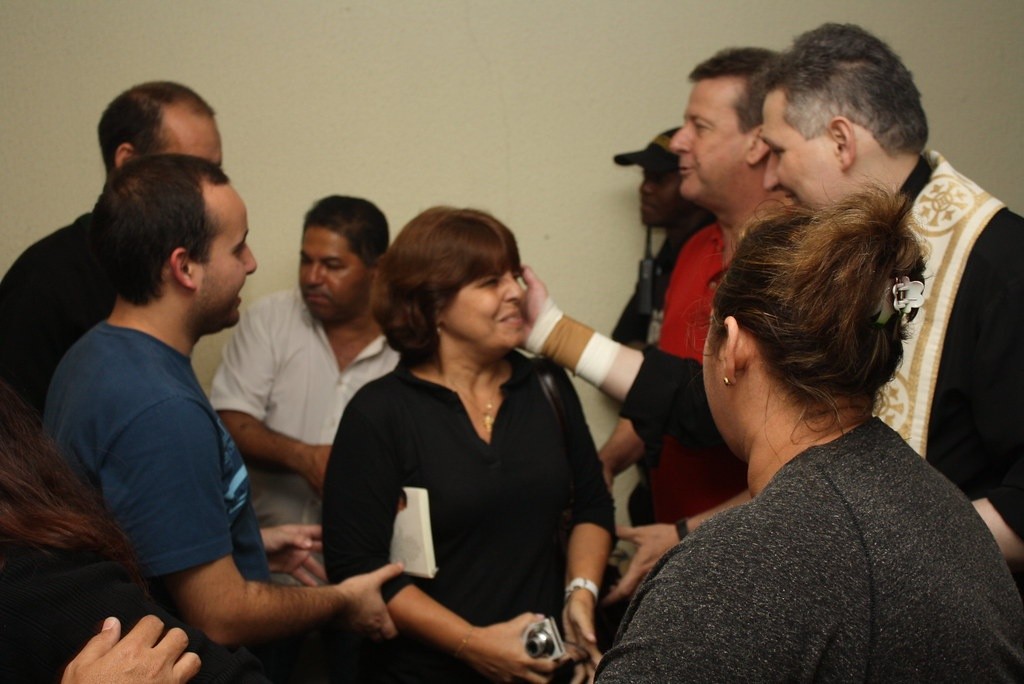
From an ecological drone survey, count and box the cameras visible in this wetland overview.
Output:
[522,616,568,661]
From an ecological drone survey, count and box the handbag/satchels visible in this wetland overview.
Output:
[530,356,630,655]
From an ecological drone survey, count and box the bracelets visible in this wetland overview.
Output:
[455,625,472,656]
[566,579,598,604]
[677,518,689,541]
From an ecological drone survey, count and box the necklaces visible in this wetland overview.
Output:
[436,363,501,433]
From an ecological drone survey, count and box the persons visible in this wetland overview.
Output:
[211,196,401,592]
[0,82,222,396]
[593,178,1024,684]
[0,384,267,684]
[41,154,404,684]
[610,126,717,530]
[598,48,798,599]
[517,24,1024,599]
[322,207,617,684]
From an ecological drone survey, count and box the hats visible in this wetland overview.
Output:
[612,127,682,175]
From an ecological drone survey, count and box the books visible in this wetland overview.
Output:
[391,487,439,579]
